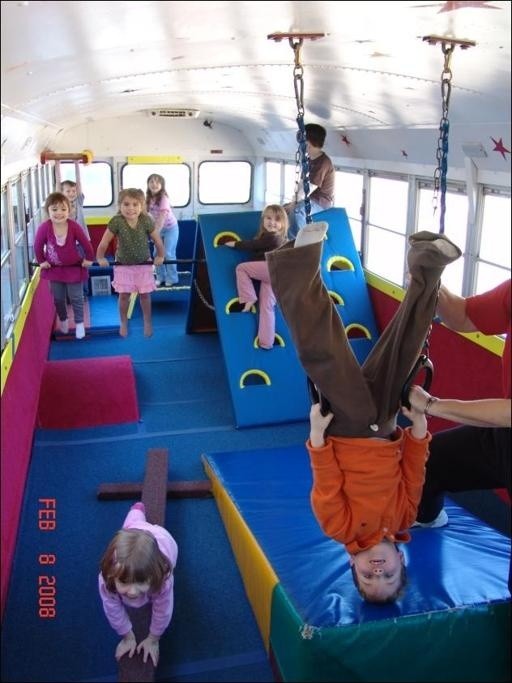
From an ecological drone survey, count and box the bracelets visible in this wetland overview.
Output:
[424,397,438,416]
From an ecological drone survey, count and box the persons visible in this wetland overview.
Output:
[145,174,180,287]
[265,222,461,605]
[283,124,334,240]
[96,189,164,337]
[224,205,289,350]
[55,159,91,301]
[99,501,179,667]
[408,279,512,597]
[34,193,94,339]
